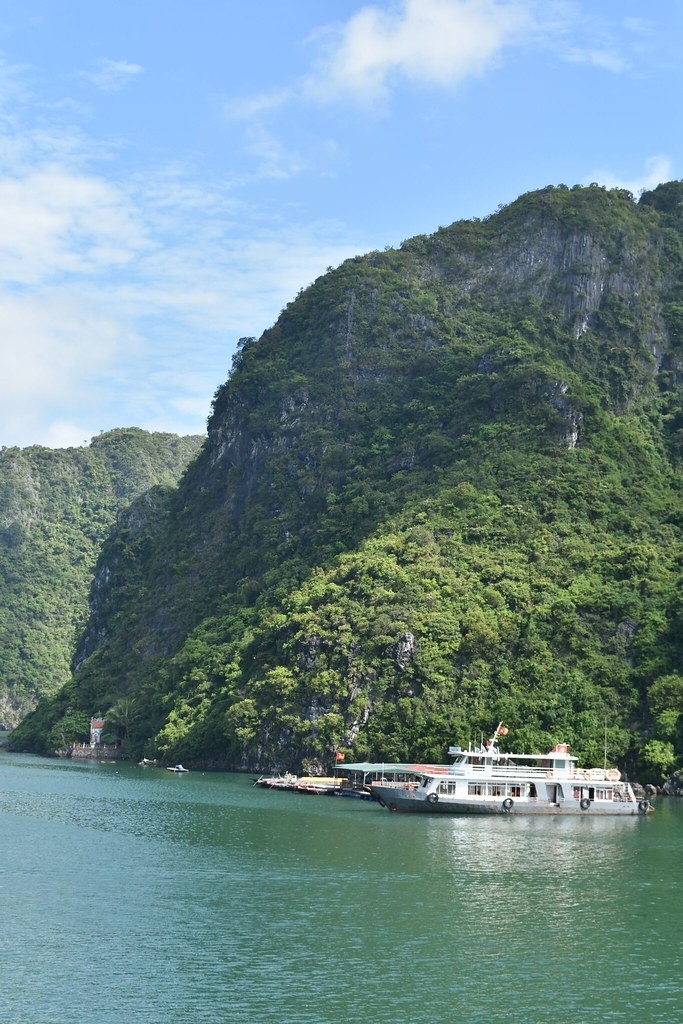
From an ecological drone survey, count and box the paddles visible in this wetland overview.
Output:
[268,778,278,790]
[174,766,176,773]
[253,775,263,787]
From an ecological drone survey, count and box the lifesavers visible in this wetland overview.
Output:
[546,771,556,779]
[639,800,649,811]
[584,772,592,780]
[427,793,438,804]
[503,798,514,809]
[580,798,591,810]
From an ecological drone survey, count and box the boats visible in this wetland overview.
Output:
[367,720,656,815]
[166,764,189,772]
[331,763,456,799]
